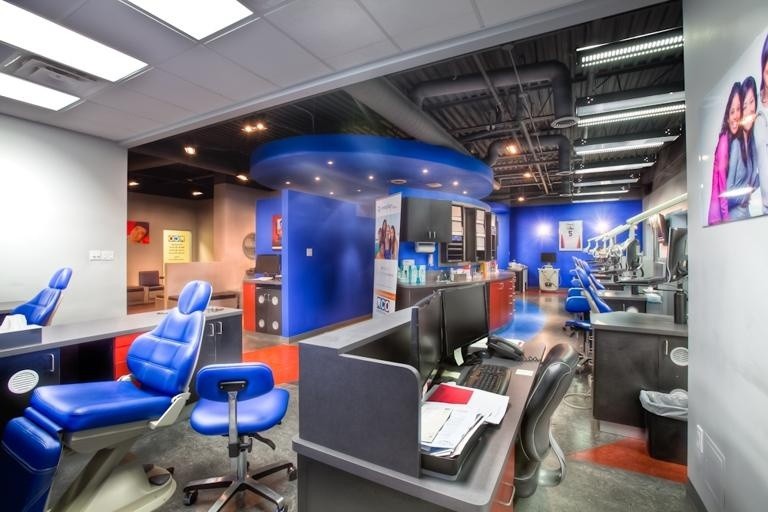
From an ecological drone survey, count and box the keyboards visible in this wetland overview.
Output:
[457,364,512,395]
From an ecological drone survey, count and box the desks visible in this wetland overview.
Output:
[291,304,546,511]
[590,272,687,439]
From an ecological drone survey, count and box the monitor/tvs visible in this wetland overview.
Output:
[541,252,555,263]
[254,254,280,276]
[442,283,491,357]
[413,292,442,391]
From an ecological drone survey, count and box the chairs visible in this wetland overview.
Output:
[138,271,163,305]
[182,360,298,512]
[563,255,612,372]
[512,344,580,499]
[6,265,73,326]
[0,277,213,512]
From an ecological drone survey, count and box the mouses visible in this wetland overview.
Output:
[473,350,489,357]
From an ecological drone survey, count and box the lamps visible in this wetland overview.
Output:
[565,24,683,205]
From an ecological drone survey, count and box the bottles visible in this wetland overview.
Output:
[674,289,687,323]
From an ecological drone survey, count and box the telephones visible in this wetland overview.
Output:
[486,336,525,362]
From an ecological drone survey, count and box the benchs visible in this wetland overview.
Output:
[166,291,239,309]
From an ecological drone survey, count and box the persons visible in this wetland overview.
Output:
[726,77,764,221]
[375,221,398,260]
[752,33,767,214]
[278,221,283,239]
[708,82,743,226]
[128,222,150,245]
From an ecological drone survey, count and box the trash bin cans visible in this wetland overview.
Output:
[639,390,688,466]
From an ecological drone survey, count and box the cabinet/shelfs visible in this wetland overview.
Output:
[0,346,61,442]
[183,313,243,407]
[489,276,517,330]
[243,282,282,336]
[399,196,451,243]
